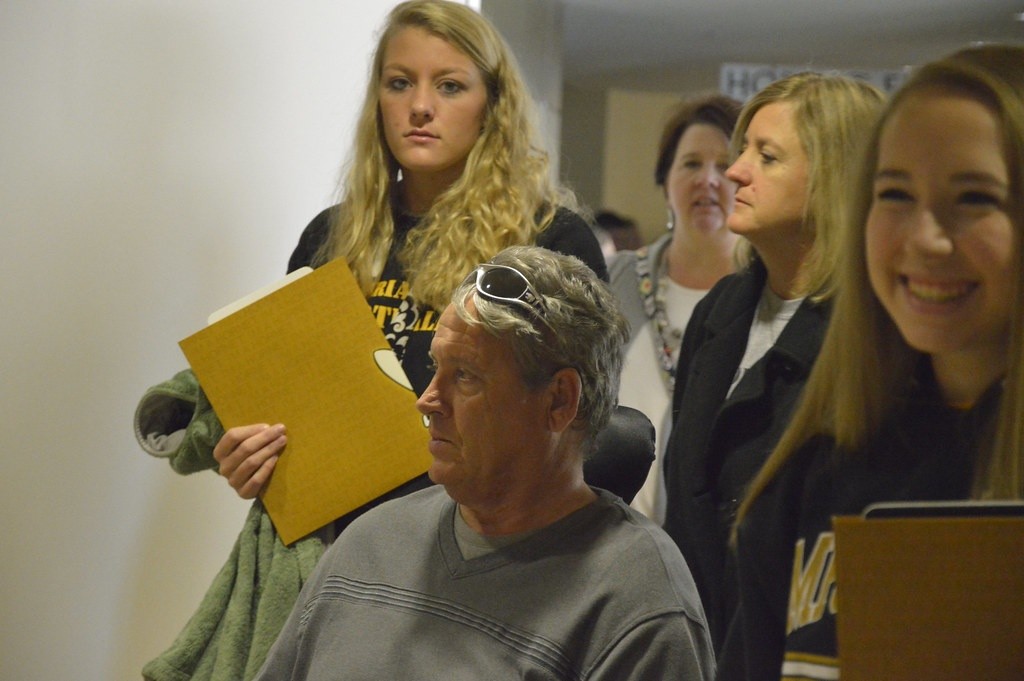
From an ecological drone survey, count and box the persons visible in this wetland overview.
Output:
[210,0,608,533]
[712,37,1024,681]
[600,93,752,530]
[657,71,892,680]
[246,244,717,681]
[591,211,640,259]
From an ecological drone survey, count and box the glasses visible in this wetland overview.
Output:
[457,262,582,377]
